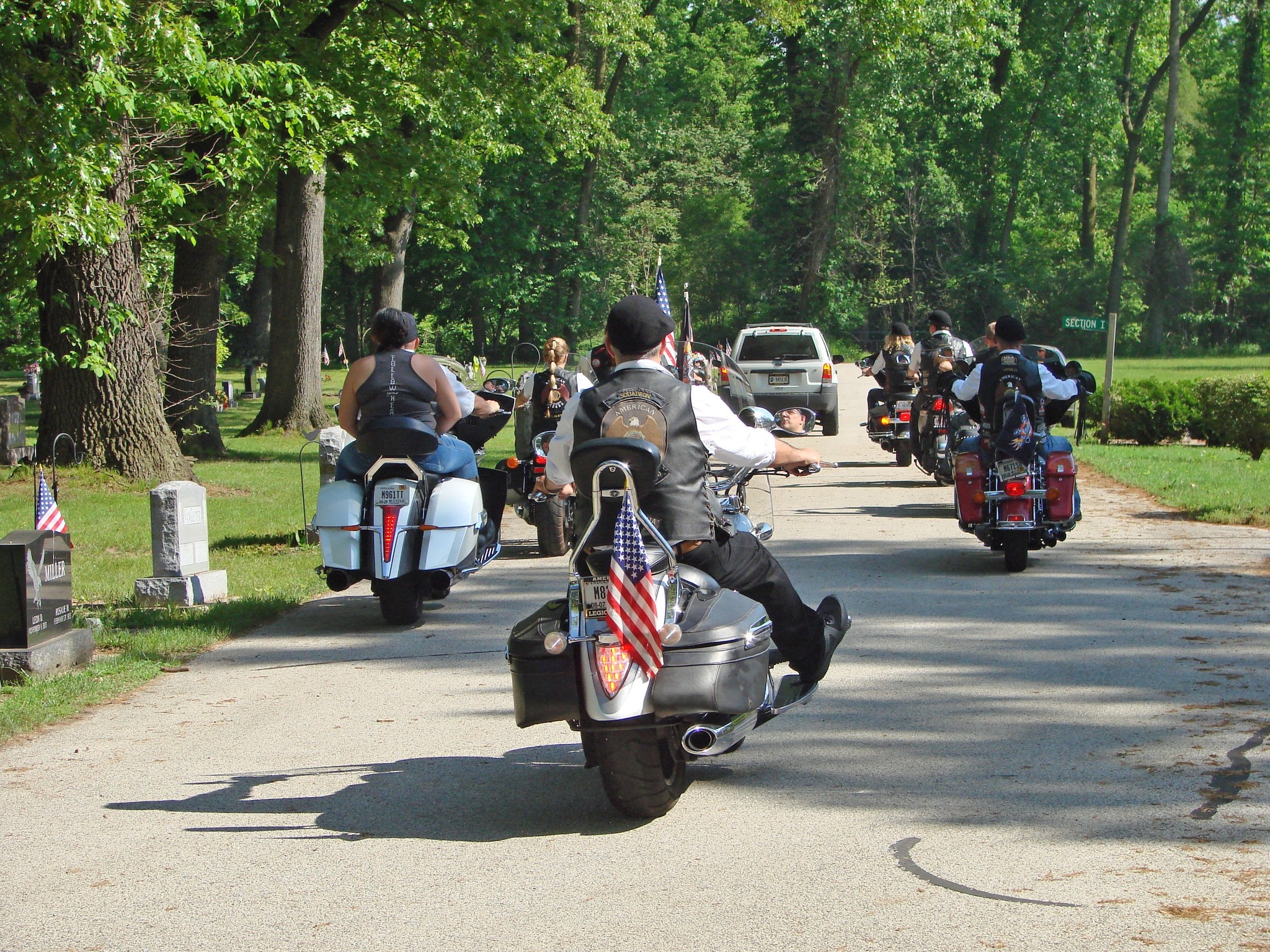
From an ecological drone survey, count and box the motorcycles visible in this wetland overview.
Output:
[495,338,854,823]
[664,350,717,391]
[493,352,600,558]
[937,348,1097,574]
[852,335,1066,488]
[298,353,518,629]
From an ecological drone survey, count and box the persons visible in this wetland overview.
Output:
[906,310,976,464]
[332,307,500,555]
[938,315,1088,521]
[532,296,849,685]
[777,407,812,435]
[861,322,921,435]
[1034,346,1049,364]
[484,380,507,393]
[511,336,596,459]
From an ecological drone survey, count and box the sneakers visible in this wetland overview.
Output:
[477,517,496,557]
[799,594,846,682]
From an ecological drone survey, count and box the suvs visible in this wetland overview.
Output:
[728,321,846,437]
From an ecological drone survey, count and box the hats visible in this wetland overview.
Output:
[892,322,911,336]
[403,312,419,345]
[929,310,952,328]
[606,295,677,354]
[995,315,1027,340]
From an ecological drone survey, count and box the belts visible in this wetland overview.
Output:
[672,539,704,556]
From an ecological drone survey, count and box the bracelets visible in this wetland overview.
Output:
[542,474,564,497]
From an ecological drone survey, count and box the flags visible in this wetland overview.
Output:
[707,339,732,373]
[680,293,695,382]
[35,464,74,550]
[654,267,679,372]
[321,344,330,366]
[605,490,664,678]
[338,338,343,357]
